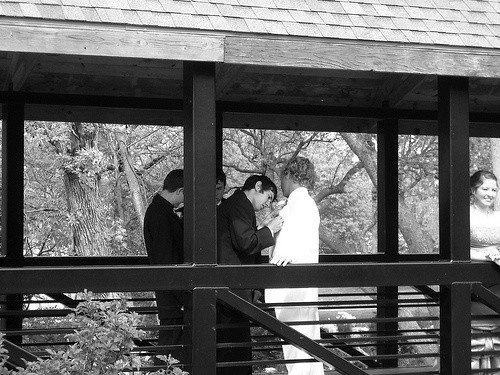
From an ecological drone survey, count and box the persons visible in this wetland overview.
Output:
[216,168,227,207]
[143,168,186,366]
[217,174,283,375]
[470,169,500,267]
[264,157,325,375]
